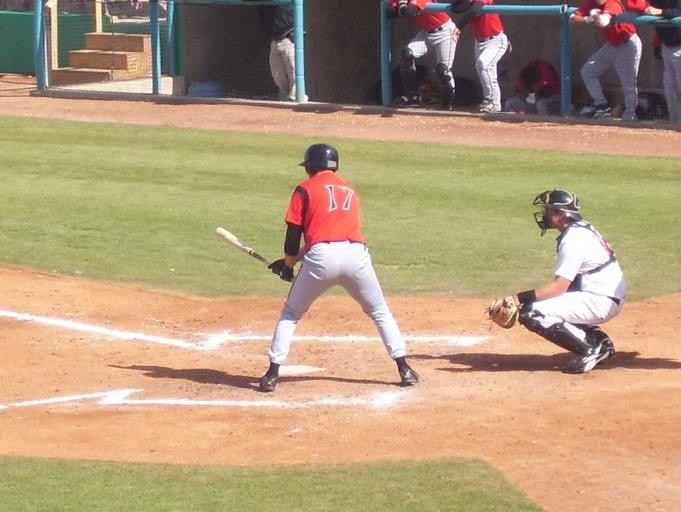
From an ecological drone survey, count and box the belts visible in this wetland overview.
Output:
[477,36,493,43]
[609,296,621,306]
[428,27,442,33]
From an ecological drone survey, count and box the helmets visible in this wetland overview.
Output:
[534,189,583,236]
[299,144,338,177]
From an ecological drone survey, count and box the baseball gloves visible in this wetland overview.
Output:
[489,295,519,328]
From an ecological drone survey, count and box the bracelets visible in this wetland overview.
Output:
[516,290,538,305]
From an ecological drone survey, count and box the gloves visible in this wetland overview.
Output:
[269,260,297,282]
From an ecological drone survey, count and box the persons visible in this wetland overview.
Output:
[503,57,561,116]
[256,141,419,391]
[256,0,310,103]
[391,48,430,102]
[622,0,680,128]
[388,0,462,112]
[482,188,626,375]
[566,1,643,123]
[449,0,511,113]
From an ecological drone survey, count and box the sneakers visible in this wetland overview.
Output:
[401,370,418,386]
[398,97,420,108]
[261,375,277,390]
[586,105,613,118]
[570,341,615,372]
[479,99,495,113]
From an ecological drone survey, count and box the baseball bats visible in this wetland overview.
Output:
[216,227,273,265]
